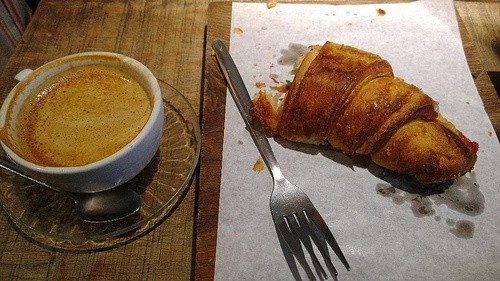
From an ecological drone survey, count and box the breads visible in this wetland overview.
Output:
[249,41,479,186]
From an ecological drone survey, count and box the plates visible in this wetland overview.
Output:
[0,77,202,241]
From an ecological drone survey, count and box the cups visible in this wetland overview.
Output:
[0,51,164,194]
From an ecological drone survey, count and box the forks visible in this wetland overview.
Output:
[211,38,351,281]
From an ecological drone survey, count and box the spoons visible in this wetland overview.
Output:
[0,156,142,223]
